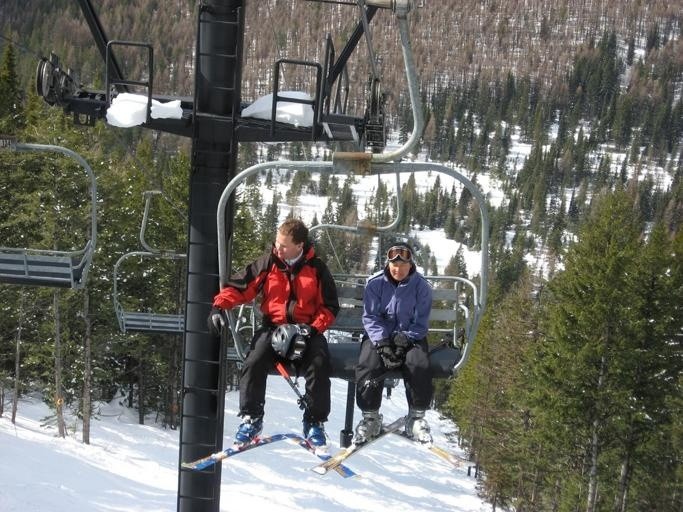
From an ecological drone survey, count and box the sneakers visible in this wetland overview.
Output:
[235,415,264,443]
[356,412,382,437]
[405,415,433,445]
[302,422,327,447]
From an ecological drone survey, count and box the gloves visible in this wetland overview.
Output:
[374,331,412,370]
[206,308,227,335]
[298,323,317,340]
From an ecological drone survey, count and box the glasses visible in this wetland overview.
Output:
[386,246,412,262]
[289,340,306,361]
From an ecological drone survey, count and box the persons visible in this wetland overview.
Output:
[207,217,341,448]
[350,240,438,446]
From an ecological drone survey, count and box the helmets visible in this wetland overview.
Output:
[269,323,307,363]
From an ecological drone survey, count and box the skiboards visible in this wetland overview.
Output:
[310,416,464,477]
[180,433,356,479]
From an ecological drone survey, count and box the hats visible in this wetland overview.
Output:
[387,242,412,261]
